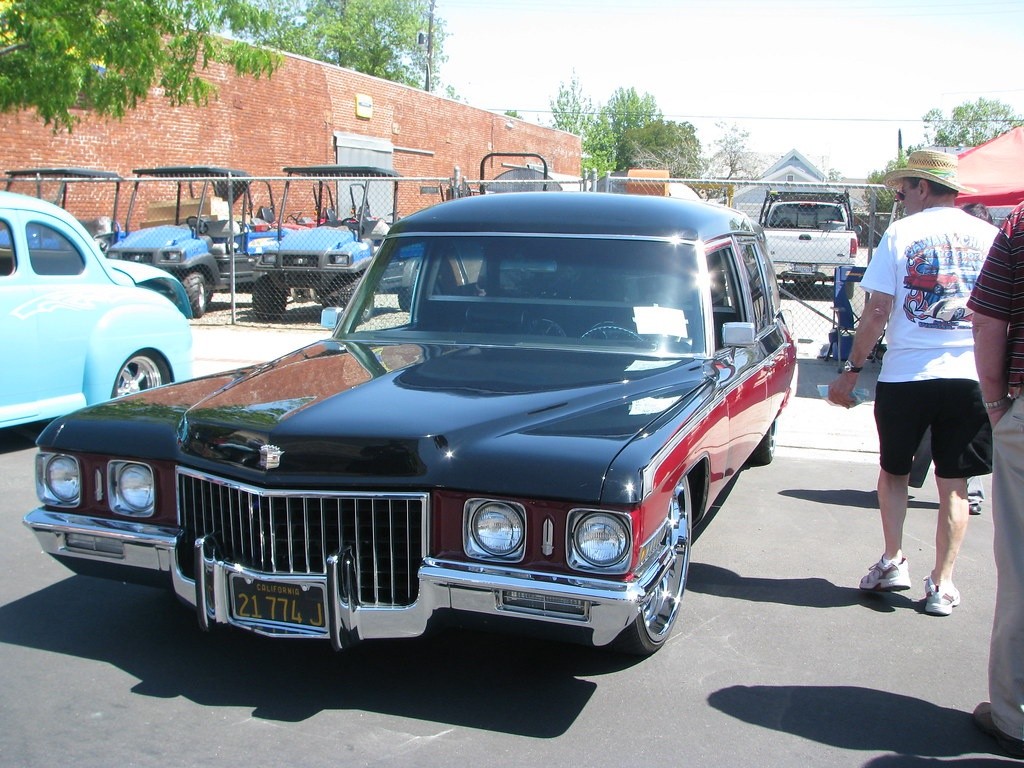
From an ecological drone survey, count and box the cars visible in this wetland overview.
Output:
[23,192,798,658]
[0,189,194,430]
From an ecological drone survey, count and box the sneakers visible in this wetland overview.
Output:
[858,554,910,591]
[922,575,960,615]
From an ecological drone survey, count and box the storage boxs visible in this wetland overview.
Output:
[627,169,671,197]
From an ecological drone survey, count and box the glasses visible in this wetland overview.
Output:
[893,185,911,201]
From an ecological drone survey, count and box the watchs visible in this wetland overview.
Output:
[844,361,863,372]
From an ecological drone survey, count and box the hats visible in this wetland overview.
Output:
[884,150,977,195]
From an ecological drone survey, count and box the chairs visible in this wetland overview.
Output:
[779,218,793,227]
[821,266,887,373]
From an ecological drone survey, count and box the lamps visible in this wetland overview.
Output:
[506,119,514,128]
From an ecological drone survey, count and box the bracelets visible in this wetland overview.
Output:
[983,398,1009,408]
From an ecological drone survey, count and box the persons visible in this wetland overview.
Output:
[829,151,1024,755]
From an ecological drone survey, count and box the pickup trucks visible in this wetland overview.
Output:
[758,191,858,298]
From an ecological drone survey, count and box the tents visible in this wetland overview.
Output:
[954,125,1024,207]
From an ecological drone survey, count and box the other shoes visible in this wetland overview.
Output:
[974,701,1024,757]
[968,503,982,515]
